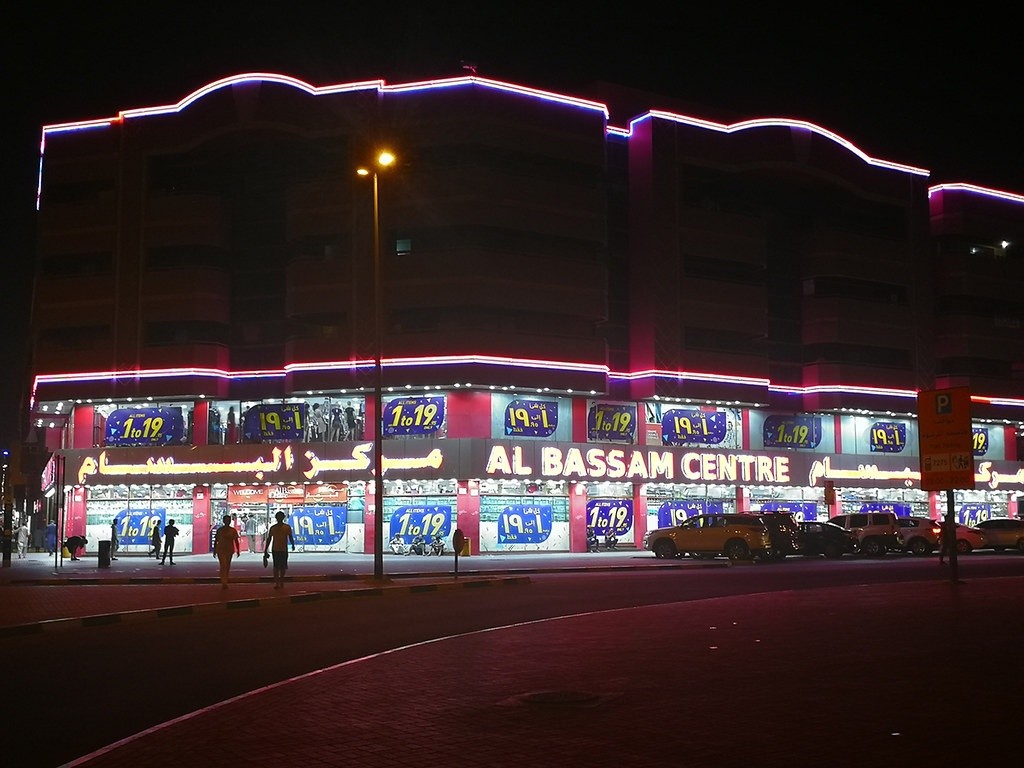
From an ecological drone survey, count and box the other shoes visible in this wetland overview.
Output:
[221,584,229,590]
[607,546,611,551]
[404,553,409,556]
[273,585,285,591]
[437,554,441,556]
[590,549,594,553]
[71,558,80,561]
[158,562,165,566]
[148,552,151,557]
[422,553,425,556]
[112,558,118,560]
[595,548,599,553]
[170,562,177,566]
[613,545,618,550]
[156,557,161,559]
[427,553,430,556]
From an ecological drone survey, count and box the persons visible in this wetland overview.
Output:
[226,406,235,427]
[243,406,252,420]
[264,511,295,589]
[1012,512,1021,520]
[649,417,656,423]
[427,536,445,556]
[213,515,241,590]
[67,535,88,561]
[158,519,179,565]
[148,520,162,559]
[12,519,56,560]
[606,528,618,550]
[390,533,405,555]
[938,516,961,565]
[404,534,426,556]
[305,401,356,442]
[111,519,119,561]
[233,512,267,554]
[586,527,600,553]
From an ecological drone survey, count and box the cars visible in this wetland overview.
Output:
[975,519,1024,553]
[939,522,988,554]
[796,520,859,559]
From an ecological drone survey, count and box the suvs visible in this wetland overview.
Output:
[898,517,944,557]
[643,512,773,562]
[830,513,901,557]
[691,511,802,561]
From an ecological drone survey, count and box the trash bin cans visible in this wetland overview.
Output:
[98,541,111,569]
[62,542,71,558]
[460,537,471,557]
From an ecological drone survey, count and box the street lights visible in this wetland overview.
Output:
[356,149,396,581]
